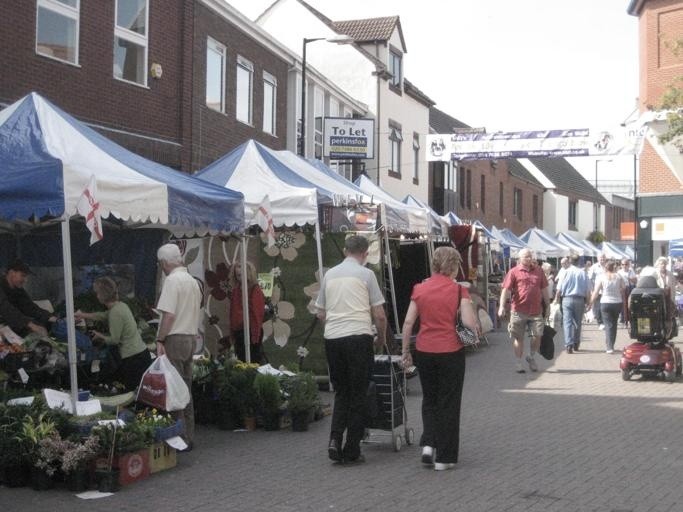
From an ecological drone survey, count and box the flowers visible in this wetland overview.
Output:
[32,427,100,476]
[134,407,175,432]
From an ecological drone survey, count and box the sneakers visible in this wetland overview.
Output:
[526,354,539,371]
[421,446,436,462]
[517,359,524,373]
[435,463,456,470]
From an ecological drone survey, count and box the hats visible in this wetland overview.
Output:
[8,259,34,275]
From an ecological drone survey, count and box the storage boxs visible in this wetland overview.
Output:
[117,449,149,484]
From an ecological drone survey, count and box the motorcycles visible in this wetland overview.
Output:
[617,276,682,383]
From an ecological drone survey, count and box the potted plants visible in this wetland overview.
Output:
[89,403,120,492]
[191,355,280,433]
[0,414,58,491]
[282,373,319,430]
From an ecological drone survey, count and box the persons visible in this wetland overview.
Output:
[496,247,682,375]
[73,277,151,389]
[154,244,200,453]
[0,262,62,337]
[400,246,478,471]
[315,235,386,465]
[229,260,268,365]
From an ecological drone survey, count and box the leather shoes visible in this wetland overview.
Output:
[345,455,366,466]
[329,439,342,460]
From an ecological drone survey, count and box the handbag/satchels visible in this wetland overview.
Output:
[456,308,480,345]
[539,319,557,359]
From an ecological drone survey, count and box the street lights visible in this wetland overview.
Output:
[594,156,614,232]
[297,33,356,161]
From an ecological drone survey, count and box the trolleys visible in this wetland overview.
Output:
[357,334,414,452]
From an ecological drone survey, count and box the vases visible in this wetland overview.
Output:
[65,472,88,490]
[153,420,180,441]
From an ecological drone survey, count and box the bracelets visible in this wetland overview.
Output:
[155,340,164,343]
